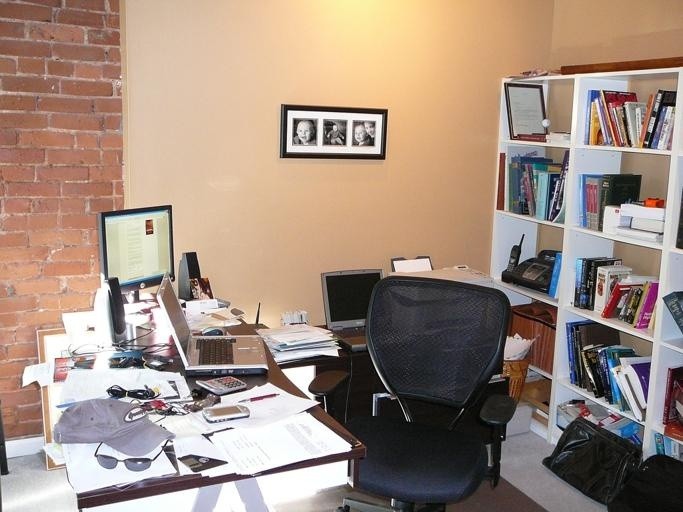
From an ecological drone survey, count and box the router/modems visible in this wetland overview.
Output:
[245,300,270,329]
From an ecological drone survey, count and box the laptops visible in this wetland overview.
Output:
[320,269,383,351]
[156,272,269,377]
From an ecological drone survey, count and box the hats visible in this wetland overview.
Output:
[54,398,175,456]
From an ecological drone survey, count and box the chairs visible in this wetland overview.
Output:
[308,276,515,512]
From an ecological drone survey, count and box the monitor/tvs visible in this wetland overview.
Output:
[99,205,176,305]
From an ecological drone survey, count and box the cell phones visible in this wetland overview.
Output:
[202,405,250,422]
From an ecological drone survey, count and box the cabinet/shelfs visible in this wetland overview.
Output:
[489,67,683,463]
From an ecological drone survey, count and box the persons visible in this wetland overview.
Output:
[327,125,344,145]
[363,121,375,138]
[297,120,315,145]
[355,124,372,146]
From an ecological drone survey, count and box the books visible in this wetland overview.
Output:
[655,433,683,462]
[53,355,96,381]
[549,253,562,300]
[536,409,549,419]
[575,257,659,330]
[518,134,570,143]
[663,365,683,441]
[566,320,651,422]
[509,150,569,223]
[663,291,683,333]
[584,88,677,150]
[580,174,642,231]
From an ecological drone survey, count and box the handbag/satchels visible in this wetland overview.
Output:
[542,416,682,511]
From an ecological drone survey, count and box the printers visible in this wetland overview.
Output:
[389,253,494,289]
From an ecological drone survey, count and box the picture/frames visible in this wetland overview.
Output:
[280,103,388,159]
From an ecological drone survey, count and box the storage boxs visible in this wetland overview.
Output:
[509,302,556,375]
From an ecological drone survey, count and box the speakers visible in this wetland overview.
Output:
[95,277,127,346]
[178,252,199,302]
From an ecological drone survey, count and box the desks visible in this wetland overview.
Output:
[36,318,366,512]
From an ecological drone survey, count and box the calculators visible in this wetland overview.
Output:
[195,376,247,396]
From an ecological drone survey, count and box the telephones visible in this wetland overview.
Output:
[502,233,563,294]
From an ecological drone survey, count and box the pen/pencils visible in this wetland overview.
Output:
[236,394,280,404]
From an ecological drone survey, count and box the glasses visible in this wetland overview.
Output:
[94,439,173,472]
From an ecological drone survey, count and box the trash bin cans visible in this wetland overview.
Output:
[503,357,530,404]
